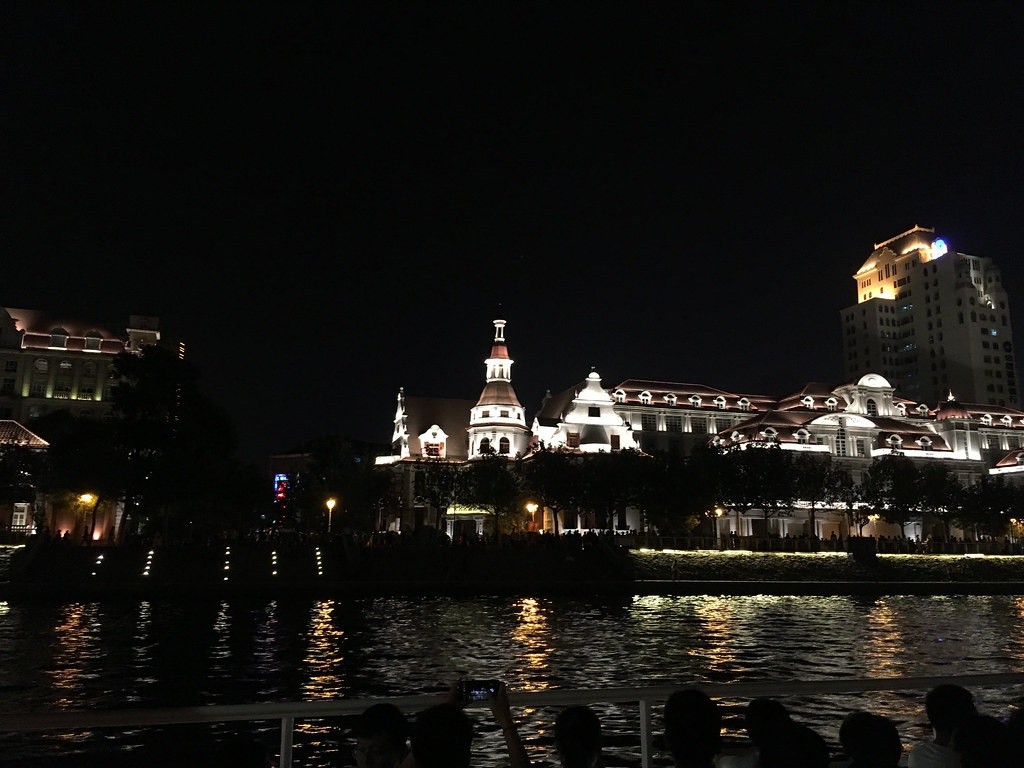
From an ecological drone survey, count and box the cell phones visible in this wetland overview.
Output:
[458,680,499,702]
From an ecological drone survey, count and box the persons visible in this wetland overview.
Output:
[352,703,409,768]
[664,689,721,768]
[399,682,530,768]
[56,529,72,540]
[555,704,603,768]
[108,526,115,544]
[209,523,1024,554]
[746,682,1024,768]
[80,526,89,546]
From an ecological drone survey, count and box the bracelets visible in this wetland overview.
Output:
[503,726,517,734]
